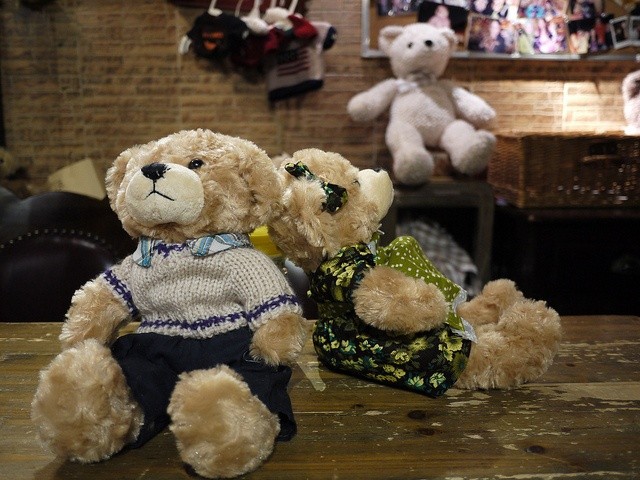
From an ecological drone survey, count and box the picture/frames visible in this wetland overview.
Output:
[362,0,640,60]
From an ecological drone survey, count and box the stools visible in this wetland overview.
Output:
[372,161,496,290]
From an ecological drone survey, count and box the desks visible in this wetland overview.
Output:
[1,316,639,478]
[509,204,639,297]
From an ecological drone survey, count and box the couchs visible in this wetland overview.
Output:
[0,187,138,322]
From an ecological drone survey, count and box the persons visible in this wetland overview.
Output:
[478,21,504,53]
[571,29,590,54]
[542,17,566,52]
[533,19,551,49]
[593,18,607,41]
[514,22,532,55]
[589,29,608,52]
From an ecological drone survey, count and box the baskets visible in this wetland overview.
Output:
[487,130,639,213]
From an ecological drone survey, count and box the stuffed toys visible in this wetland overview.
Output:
[266,149,562,399]
[347,22,497,184]
[29,128,311,477]
